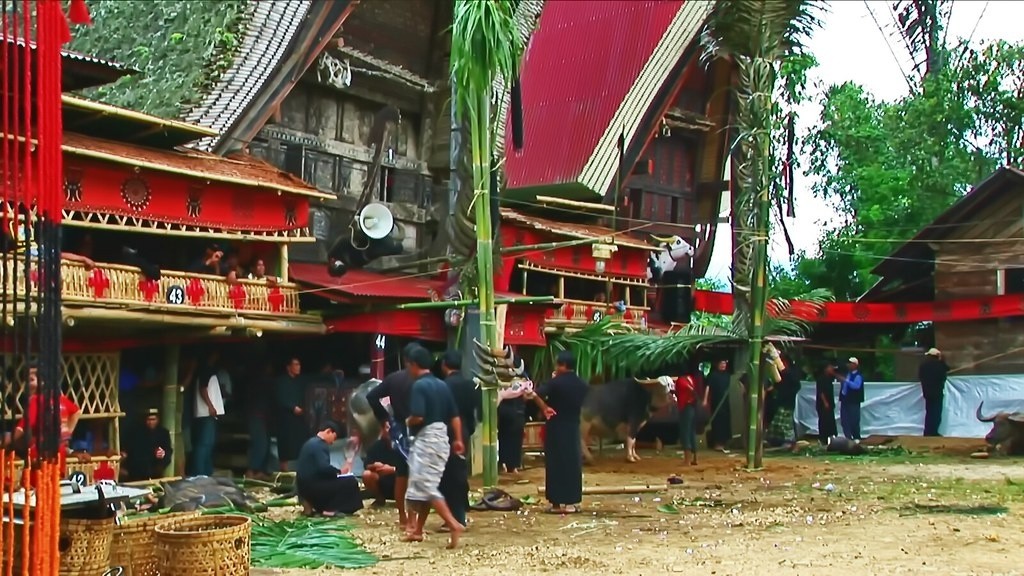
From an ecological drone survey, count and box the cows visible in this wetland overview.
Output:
[581,375,677,464]
[976,401,1024,455]
[648,234,695,281]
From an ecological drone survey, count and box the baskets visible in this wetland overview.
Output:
[113,510,203,576]
[60,511,117,576]
[155,514,252,576]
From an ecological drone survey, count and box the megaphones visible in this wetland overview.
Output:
[357,202,394,240]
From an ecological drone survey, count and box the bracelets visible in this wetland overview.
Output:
[393,467,395,472]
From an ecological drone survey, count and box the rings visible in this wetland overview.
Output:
[162,454,165,458]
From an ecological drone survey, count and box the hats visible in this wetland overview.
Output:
[144,408,160,416]
[849,357,858,365]
[925,348,942,357]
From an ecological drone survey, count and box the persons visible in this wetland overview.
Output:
[593,292,606,302]
[2,222,266,284]
[815,357,864,450]
[328,254,344,268]
[387,175,392,202]
[919,347,947,437]
[0,342,589,549]
[669,350,801,467]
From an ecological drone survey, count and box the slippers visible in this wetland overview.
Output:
[547,506,562,514]
[564,505,583,512]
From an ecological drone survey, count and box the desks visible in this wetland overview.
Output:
[5,485,156,521]
